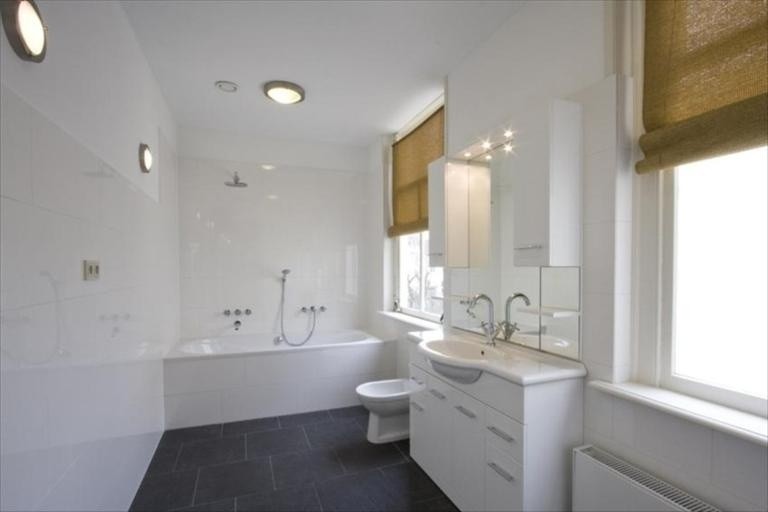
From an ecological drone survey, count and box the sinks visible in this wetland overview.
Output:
[418,339,505,385]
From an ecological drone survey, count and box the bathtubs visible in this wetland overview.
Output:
[164,327,398,433]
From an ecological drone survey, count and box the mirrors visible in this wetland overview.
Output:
[448,130,579,363]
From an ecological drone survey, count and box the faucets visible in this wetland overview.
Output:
[504,292,530,329]
[469,293,494,333]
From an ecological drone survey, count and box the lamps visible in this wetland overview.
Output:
[139,143,152,173]
[0,0,48,64]
[264,81,304,106]
[226,172,246,187]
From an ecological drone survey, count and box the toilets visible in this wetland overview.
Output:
[354,330,450,445]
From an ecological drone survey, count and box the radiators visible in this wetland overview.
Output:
[573,442,725,512]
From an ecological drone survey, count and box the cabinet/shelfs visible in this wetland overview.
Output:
[467,164,492,268]
[425,373,483,512]
[407,362,425,474]
[515,98,586,269]
[483,403,584,512]
[426,152,469,267]
